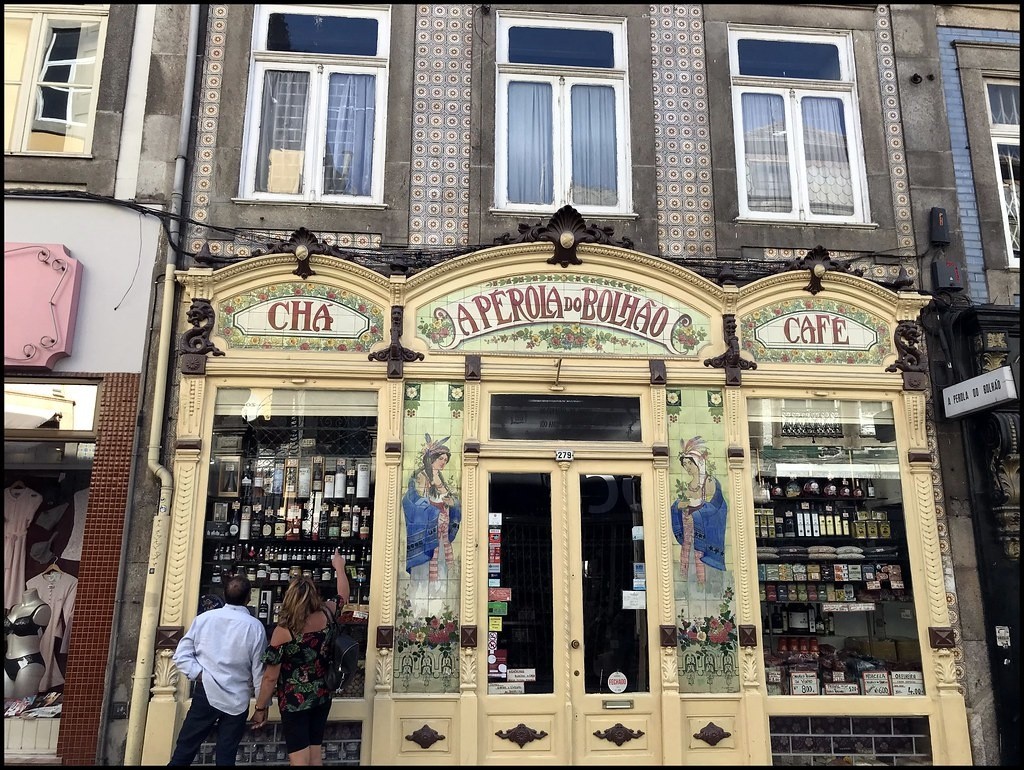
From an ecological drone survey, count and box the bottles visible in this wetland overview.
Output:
[761,602,835,637]
[754,476,875,537]
[205,456,376,629]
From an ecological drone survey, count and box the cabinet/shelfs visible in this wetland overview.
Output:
[192,444,934,765]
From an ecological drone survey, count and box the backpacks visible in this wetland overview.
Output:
[321,604,359,695]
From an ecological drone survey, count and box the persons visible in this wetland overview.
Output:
[6,588,51,703]
[166,575,272,768]
[249,547,351,767]
